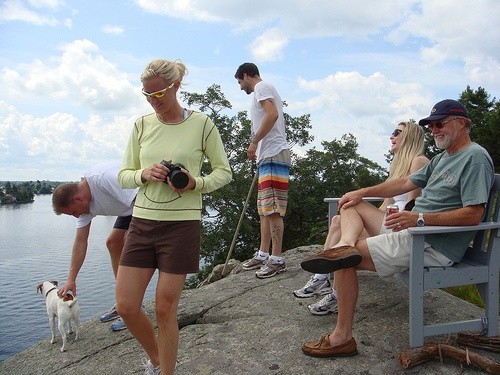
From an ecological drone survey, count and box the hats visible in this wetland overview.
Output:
[419,99,468,127]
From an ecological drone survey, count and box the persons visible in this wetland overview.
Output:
[292,123,429,315]
[45,168,138,331]
[302,99,494,360]
[113,60,232,375]
[230,60,290,279]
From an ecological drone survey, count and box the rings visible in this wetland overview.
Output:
[397,223,401,228]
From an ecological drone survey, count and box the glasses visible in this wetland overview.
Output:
[428,117,461,130]
[141,82,175,99]
[392,129,405,137]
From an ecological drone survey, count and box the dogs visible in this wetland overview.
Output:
[36,280,80,353]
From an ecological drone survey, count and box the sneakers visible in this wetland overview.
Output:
[307,288,339,316]
[242,252,270,271]
[111,304,148,331]
[100,303,120,323]
[292,273,333,300]
[254,255,288,279]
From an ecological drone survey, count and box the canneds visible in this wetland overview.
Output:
[386,205,399,217]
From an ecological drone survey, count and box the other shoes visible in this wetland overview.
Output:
[141,356,160,375]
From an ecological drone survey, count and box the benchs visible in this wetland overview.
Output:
[324,173,500,346]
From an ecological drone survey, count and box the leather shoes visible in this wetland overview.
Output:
[301,334,358,358]
[299,245,362,273]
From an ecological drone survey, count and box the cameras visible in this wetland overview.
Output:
[160,160,189,189]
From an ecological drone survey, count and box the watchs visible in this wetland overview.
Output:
[416,213,425,227]
